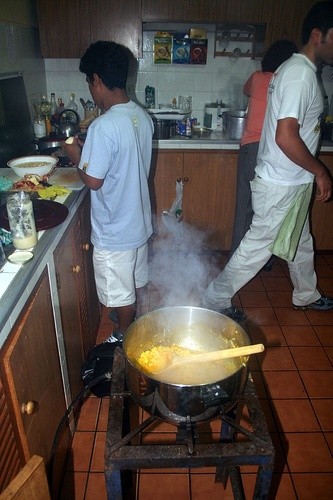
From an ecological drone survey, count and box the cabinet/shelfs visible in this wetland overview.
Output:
[0,191,101,500]
[265,0,322,56]
[35,0,143,59]
[141,0,271,25]
[213,25,255,59]
[308,152,333,254]
[148,148,240,256]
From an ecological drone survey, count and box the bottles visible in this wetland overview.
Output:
[35,93,78,138]
[7,193,38,249]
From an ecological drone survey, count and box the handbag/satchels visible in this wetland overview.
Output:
[81,342,125,398]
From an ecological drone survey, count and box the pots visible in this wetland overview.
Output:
[218,109,248,139]
[122,306,251,426]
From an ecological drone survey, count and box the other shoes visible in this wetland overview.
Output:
[110,310,121,323]
[102,330,123,344]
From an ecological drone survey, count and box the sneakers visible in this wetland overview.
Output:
[221,307,245,323]
[292,295,333,311]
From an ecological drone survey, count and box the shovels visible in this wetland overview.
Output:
[153,342,265,375]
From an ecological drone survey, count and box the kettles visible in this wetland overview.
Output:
[51,109,81,138]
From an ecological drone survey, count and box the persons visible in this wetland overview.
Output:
[196,0,333,319]
[61,39,152,342]
[228,39,300,271]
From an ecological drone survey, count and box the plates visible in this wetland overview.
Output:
[0,199,68,231]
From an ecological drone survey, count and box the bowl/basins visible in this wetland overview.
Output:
[7,155,59,178]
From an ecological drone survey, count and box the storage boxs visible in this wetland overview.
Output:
[154,28,208,65]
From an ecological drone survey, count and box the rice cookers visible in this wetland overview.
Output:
[205,99,230,132]
